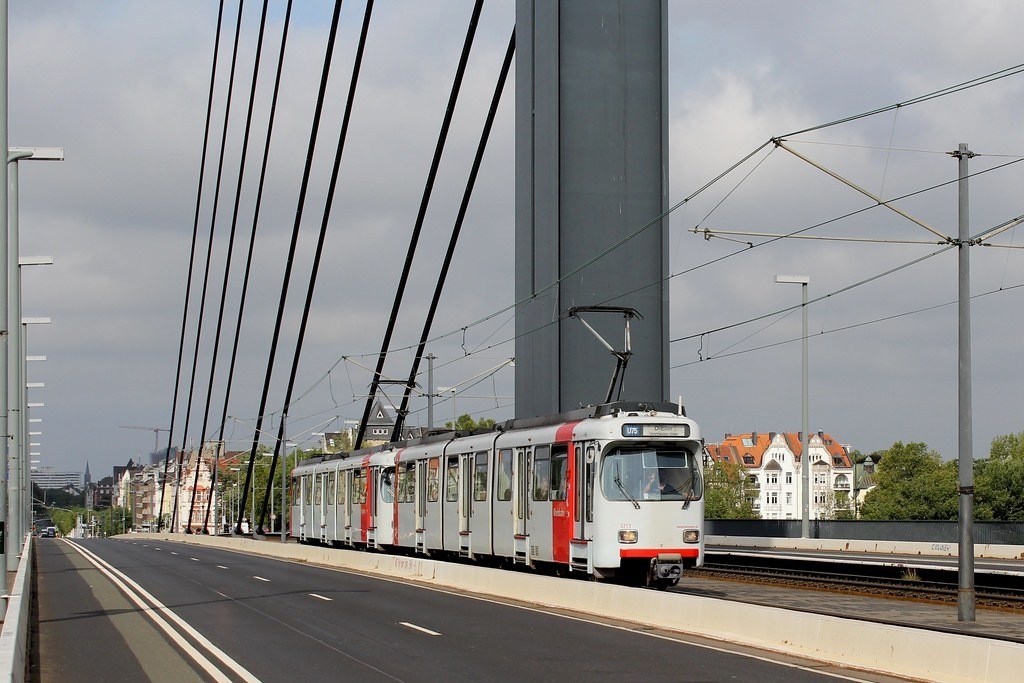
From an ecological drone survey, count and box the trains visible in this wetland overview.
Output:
[290,395,705,590]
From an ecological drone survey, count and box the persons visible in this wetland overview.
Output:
[643,471,689,497]
[223,522,230,533]
[56,531,60,537]
[196,528,202,535]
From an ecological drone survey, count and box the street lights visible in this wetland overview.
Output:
[19,257,55,557]
[5,146,64,572]
[263,453,274,533]
[245,461,255,528]
[344,420,360,445]
[437,386,456,430]
[775,275,810,538]
[286,444,297,469]
[312,432,325,454]
[230,468,240,521]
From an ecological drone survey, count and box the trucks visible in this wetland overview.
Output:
[46,526,57,538]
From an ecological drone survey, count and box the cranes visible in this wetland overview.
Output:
[119,426,170,452]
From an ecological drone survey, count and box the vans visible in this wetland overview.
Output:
[40,529,48,538]
